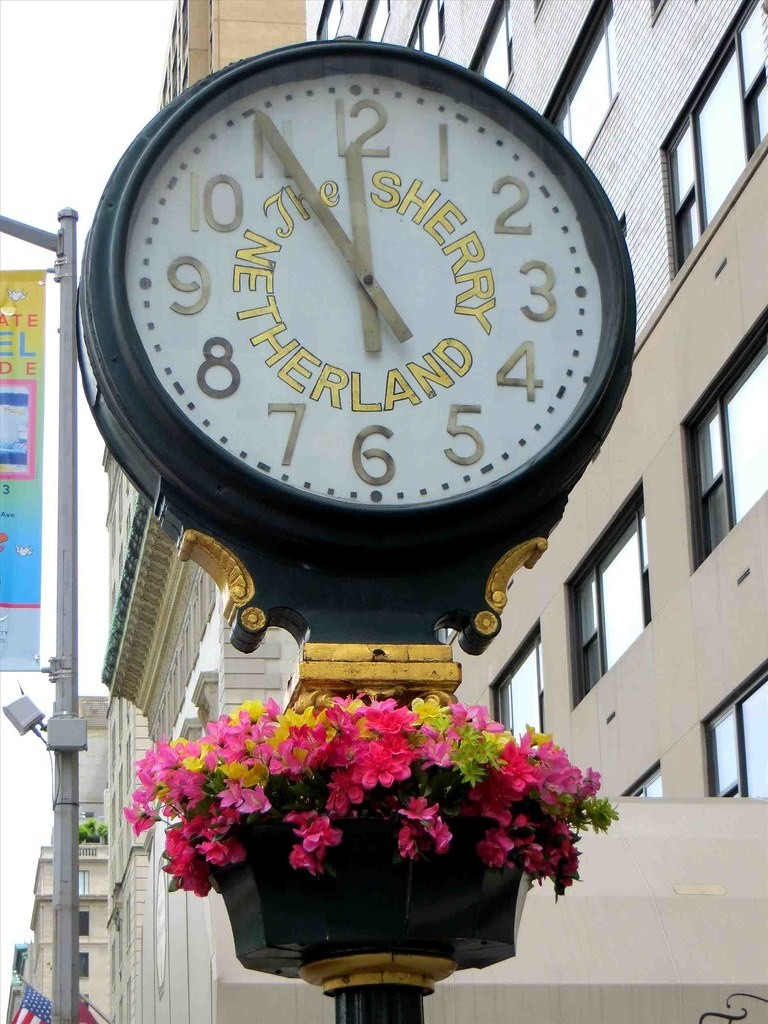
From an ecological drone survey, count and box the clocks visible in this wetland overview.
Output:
[73,34,639,664]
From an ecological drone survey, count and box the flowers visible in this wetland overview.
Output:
[119,692,619,896]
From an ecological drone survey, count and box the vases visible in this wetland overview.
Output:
[204,821,532,977]
